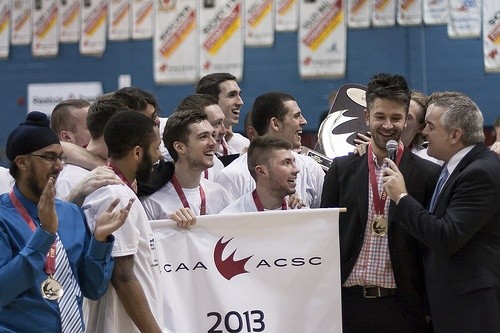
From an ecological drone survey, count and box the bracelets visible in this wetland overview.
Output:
[396,193,407,206]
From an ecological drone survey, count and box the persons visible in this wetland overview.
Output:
[382,90,499,333]
[0,72,500,333]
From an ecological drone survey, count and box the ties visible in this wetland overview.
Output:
[430,169,449,215]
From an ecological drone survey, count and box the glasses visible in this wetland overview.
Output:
[29,154,67,163]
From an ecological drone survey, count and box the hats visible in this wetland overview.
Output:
[6,111,60,161]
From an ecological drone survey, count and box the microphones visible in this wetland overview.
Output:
[386,140,398,176]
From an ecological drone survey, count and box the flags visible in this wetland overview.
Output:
[150,207,342,333]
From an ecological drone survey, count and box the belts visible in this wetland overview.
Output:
[342,285,398,298]
